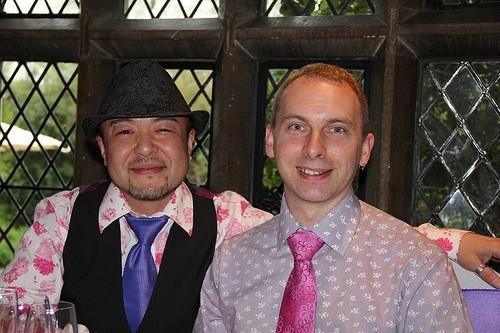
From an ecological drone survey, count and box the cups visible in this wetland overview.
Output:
[17,295,52,333]
[24,301,78,333]
[0,289,19,333]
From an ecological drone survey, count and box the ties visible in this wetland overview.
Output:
[119,214,170,331]
[275,229,325,333]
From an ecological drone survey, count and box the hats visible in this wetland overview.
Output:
[82,59,210,142]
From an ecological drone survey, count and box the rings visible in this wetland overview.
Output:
[475,264,486,274]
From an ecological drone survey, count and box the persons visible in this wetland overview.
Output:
[0,60,500,333]
[192,64,474,333]
